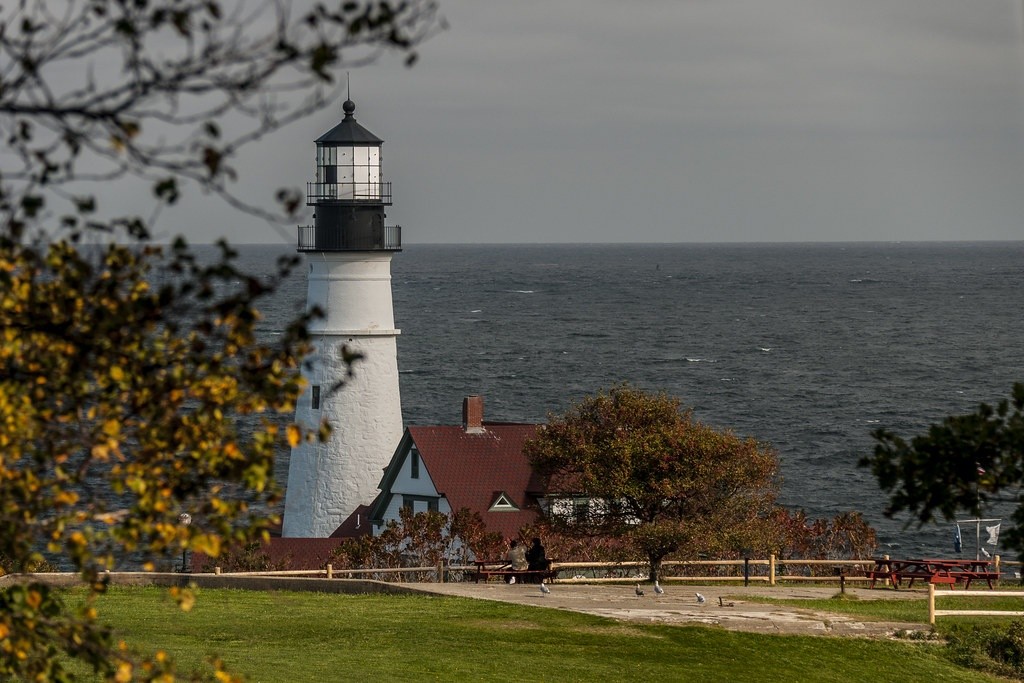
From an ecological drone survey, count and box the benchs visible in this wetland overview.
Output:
[972,572,1008,580]
[858,570,940,583]
[479,570,548,580]
[907,571,969,584]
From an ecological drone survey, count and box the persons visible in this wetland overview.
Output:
[504,541,529,585]
[528,536,547,584]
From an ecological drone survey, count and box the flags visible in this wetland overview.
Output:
[986,524,1000,546]
[976,462,995,484]
[952,526,963,552]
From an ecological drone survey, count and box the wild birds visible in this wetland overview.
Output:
[654,581,664,596]
[979,548,991,560]
[541,583,551,597]
[510,576,516,584]
[636,584,644,595]
[694,593,705,603]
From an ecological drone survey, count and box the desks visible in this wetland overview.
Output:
[474,559,554,584]
[869,560,959,590]
[958,560,994,590]
